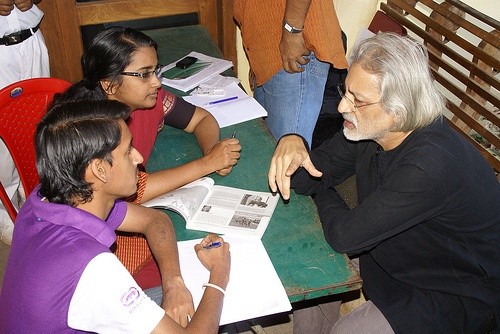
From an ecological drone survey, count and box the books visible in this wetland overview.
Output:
[138,176,281,241]
[182,82,269,129]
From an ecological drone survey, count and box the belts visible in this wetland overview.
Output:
[0,25,39,46]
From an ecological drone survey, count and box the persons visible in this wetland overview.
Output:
[0,98,232,334]
[46,25,242,205]
[234,0,348,155]
[0,0,49,247]
[269,31,500,334]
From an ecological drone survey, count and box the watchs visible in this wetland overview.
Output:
[282,17,304,33]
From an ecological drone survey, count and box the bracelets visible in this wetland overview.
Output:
[202,282,226,297]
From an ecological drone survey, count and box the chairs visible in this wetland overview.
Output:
[0,77,76,225]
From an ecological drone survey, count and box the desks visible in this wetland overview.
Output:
[143,25,364,334]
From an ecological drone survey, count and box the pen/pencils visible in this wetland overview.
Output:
[203,241,221,249]
[232,131,237,138]
[206,96,238,104]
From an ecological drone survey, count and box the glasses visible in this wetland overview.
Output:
[120,64,161,82]
[337,82,379,110]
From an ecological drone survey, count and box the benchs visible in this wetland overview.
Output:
[367,0,500,185]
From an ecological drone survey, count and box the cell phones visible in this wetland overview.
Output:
[176,57,197,69]
